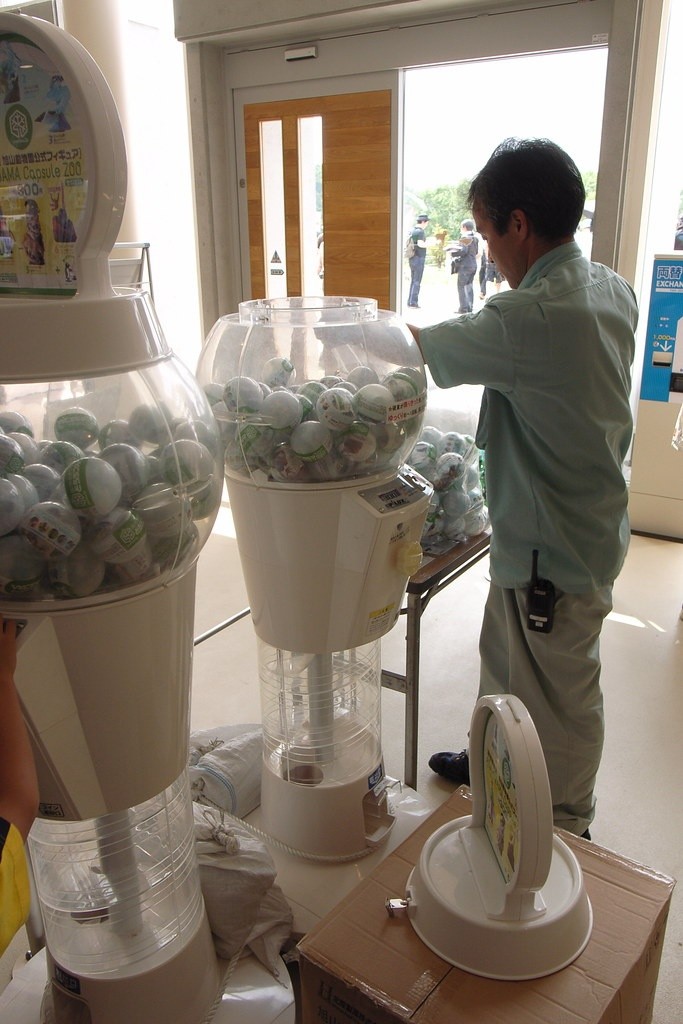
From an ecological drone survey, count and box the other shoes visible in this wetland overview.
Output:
[579,827,591,841]
[480,293,485,300]
[454,310,472,313]
[429,751,469,784]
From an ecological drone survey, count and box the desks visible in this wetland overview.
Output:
[290,525,494,791]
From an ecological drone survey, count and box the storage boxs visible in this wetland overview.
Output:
[295,784,678,1024]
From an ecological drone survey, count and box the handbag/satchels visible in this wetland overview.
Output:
[449,252,459,274]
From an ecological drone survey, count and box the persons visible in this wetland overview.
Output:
[311,136,639,841]
[0,67,77,266]
[316,215,683,315]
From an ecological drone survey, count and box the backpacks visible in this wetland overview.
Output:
[403,228,420,258]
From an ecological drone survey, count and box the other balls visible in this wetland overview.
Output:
[397,414,497,558]
[0,402,224,614]
[199,348,430,488]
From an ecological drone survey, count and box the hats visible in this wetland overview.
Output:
[416,214,430,221]
